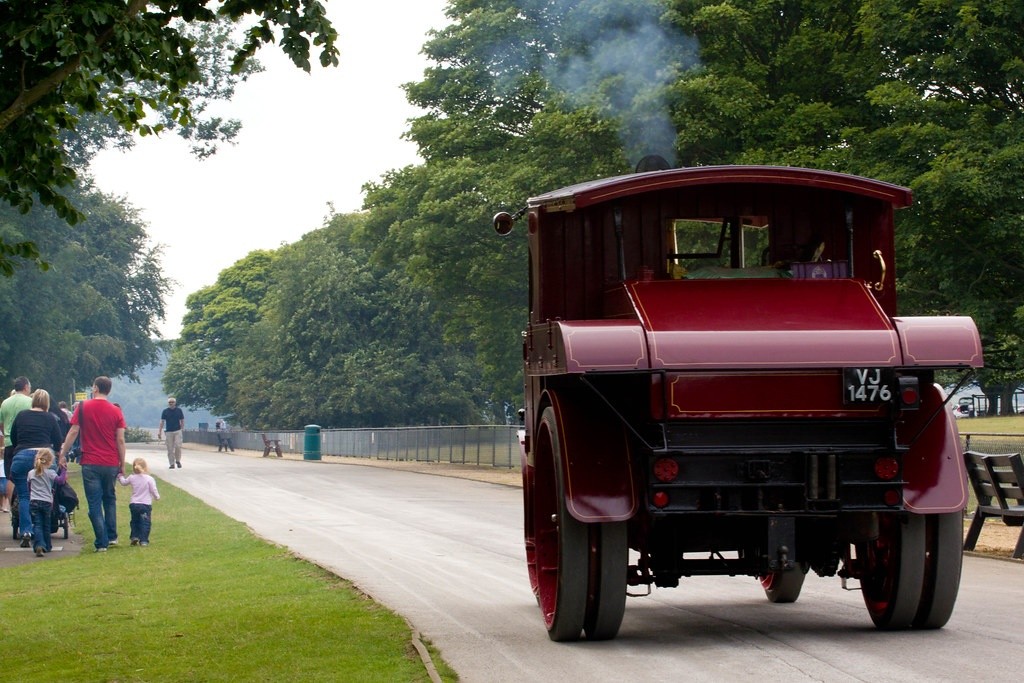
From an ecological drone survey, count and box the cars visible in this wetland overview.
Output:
[952,403,977,418]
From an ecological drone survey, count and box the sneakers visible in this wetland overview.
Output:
[95,538,117,551]
[130,537,148,546]
[20,532,31,547]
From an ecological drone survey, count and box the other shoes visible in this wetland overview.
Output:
[36,546,51,557]
[169,461,182,469]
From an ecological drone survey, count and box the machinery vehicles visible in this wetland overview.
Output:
[492,153,985,644]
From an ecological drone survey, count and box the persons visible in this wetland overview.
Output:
[216,420,236,452]
[0,377,160,558]
[158,398,185,469]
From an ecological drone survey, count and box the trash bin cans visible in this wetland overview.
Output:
[303,425,322,460]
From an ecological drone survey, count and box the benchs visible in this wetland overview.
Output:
[217,434,234,452]
[962,450,1024,559]
[262,433,283,457]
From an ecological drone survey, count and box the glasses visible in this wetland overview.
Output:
[169,402,174,403]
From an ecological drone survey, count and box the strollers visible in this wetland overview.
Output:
[4,445,69,538]
